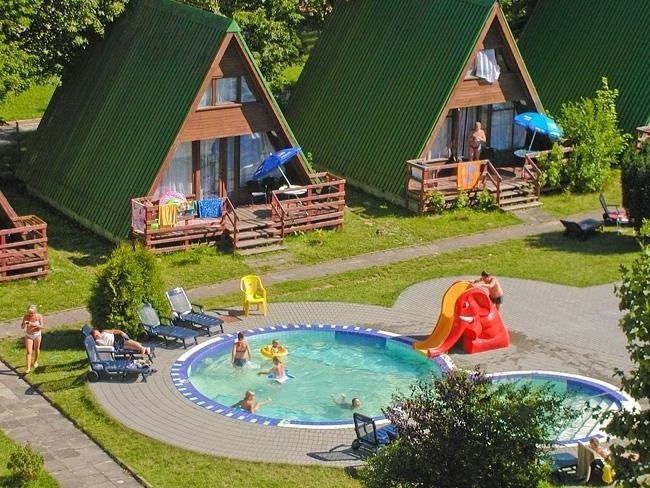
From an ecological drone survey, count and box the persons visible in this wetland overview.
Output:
[231,390,272,414]
[271,339,281,354]
[589,437,639,461]
[467,122,486,161]
[257,356,288,379]
[231,332,252,368]
[91,329,150,355]
[471,270,504,312]
[330,394,361,410]
[21,304,45,374]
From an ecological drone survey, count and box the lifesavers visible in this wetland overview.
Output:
[261,344,288,358]
[167,199,184,218]
[159,192,189,218]
[266,368,295,385]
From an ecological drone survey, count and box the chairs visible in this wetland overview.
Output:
[84,272,225,385]
[484,146,503,169]
[544,440,628,485]
[241,274,269,319]
[351,412,400,460]
[559,191,635,243]
[247,175,283,208]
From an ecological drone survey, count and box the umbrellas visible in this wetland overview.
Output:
[513,112,562,152]
[252,147,309,216]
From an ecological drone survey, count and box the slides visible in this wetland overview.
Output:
[412,315,467,360]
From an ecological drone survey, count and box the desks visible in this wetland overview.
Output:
[277,184,307,220]
[514,147,537,166]
